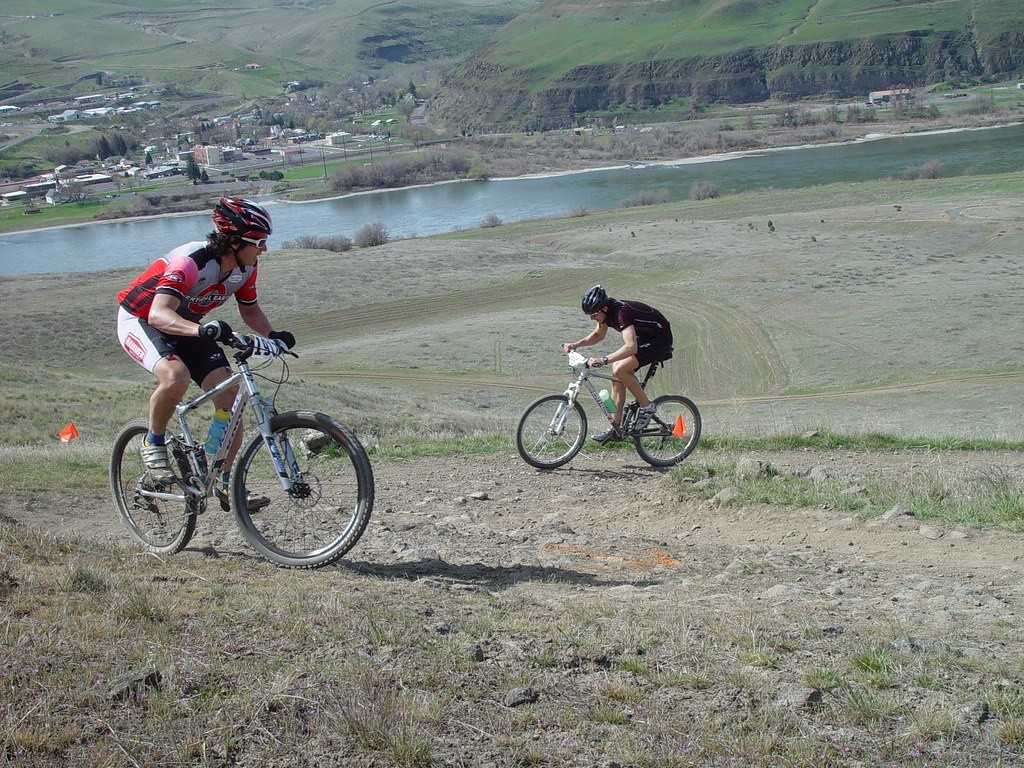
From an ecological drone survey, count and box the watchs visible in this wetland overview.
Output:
[603,356,608,365]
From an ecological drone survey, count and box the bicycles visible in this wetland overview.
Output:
[106,324,375,570]
[515,342,703,469]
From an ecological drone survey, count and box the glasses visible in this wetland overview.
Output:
[226,235,268,248]
[589,310,601,318]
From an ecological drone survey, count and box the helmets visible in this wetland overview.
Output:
[212,198,273,235]
[582,284,608,314]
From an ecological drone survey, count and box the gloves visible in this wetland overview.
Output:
[198,320,232,345]
[268,331,296,350]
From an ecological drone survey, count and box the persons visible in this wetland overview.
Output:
[565,285,674,442]
[117,196,295,510]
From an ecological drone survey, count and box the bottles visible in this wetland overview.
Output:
[205,408,231,455]
[599,389,617,413]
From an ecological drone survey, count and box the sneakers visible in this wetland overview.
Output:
[633,402,656,431]
[220,488,270,514]
[591,429,622,442]
[139,435,176,484]
